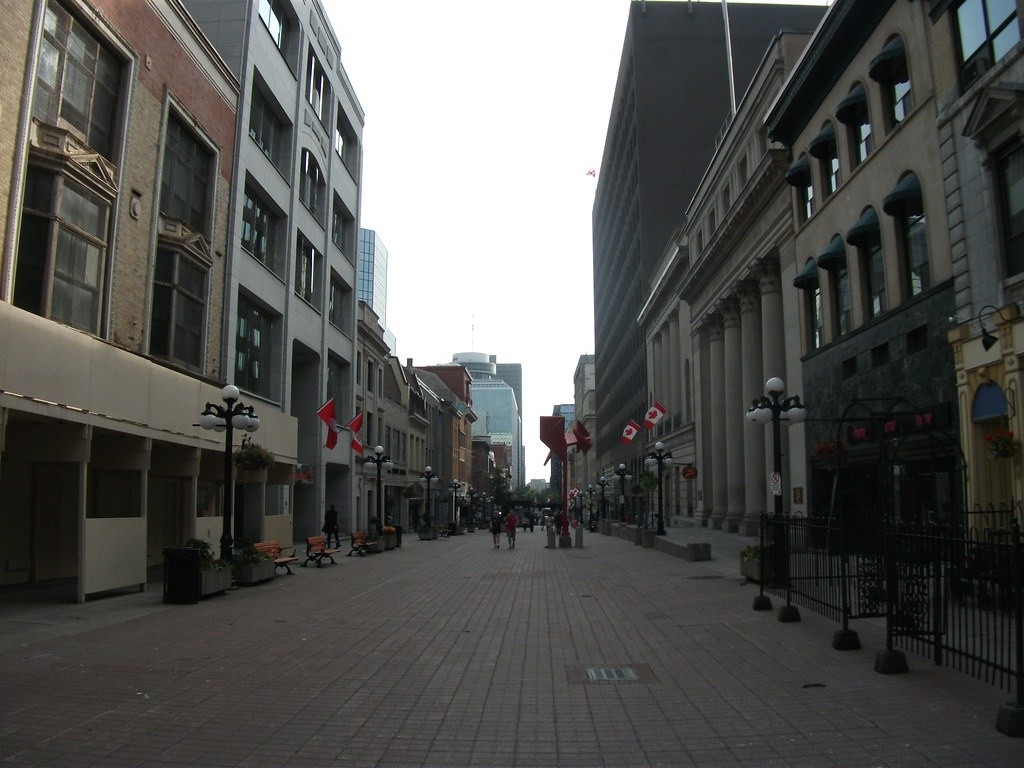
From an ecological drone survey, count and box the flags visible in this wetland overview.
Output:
[316,398,338,449]
[622,420,642,445]
[642,401,670,430]
[348,412,363,454]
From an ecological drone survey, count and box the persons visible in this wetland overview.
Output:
[596,508,599,522]
[589,511,595,532]
[527,510,535,532]
[522,514,529,532]
[540,512,545,529]
[490,510,507,548]
[503,511,518,549]
[554,510,563,536]
[322,503,342,549]
[545,514,553,536]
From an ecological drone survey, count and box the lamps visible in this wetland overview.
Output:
[978,305,1024,352]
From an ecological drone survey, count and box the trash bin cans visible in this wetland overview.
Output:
[448,522,457,536]
[394,525,402,548]
[162,546,201,604]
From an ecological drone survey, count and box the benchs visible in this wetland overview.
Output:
[298,536,341,568]
[252,540,302,575]
[345,531,378,557]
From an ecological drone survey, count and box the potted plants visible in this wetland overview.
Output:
[364,532,385,552]
[233,442,276,471]
[191,560,233,598]
[416,526,440,540]
[739,544,776,581]
[235,548,276,587]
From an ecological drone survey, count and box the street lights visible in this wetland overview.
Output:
[645,441,675,536]
[745,376,808,576]
[363,445,394,534]
[199,384,261,561]
[575,462,632,523]
[448,479,491,526]
[418,466,439,526]
